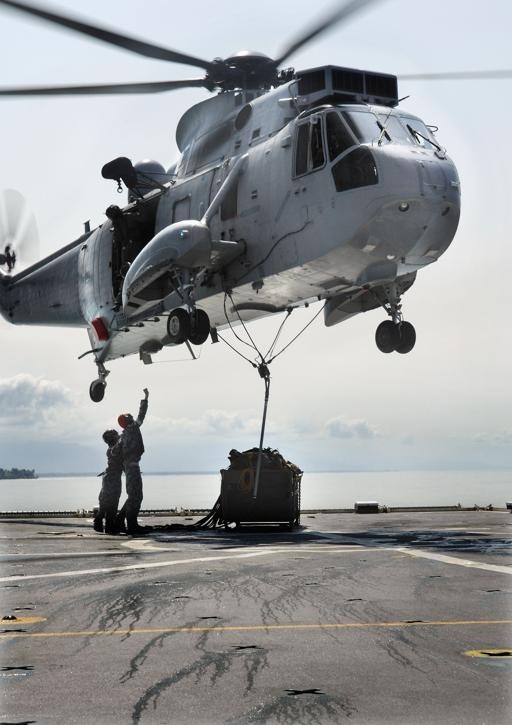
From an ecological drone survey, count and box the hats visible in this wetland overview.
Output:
[118,415,128,428]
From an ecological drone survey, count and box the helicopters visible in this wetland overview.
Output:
[0,0,511,403]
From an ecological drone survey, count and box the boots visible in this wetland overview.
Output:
[94,514,145,536]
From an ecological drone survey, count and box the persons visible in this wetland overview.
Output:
[104,203,152,306]
[92,427,124,534]
[110,387,150,532]
